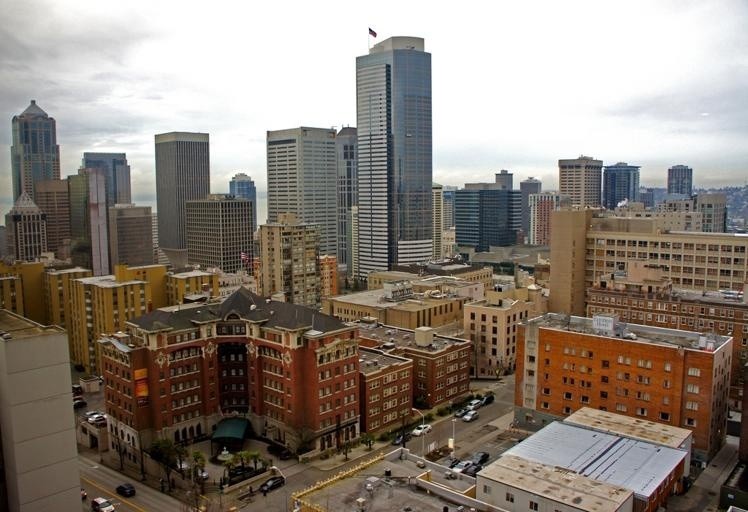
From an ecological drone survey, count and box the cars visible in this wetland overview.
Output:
[392,431,412,446]
[454,394,496,422]
[81,487,87,500]
[450,451,490,478]
[71,384,107,429]
[176,460,286,495]
[90,497,115,512]
[410,423,434,437]
[115,483,137,498]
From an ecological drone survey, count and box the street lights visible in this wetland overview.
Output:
[451,419,457,462]
[410,407,425,457]
